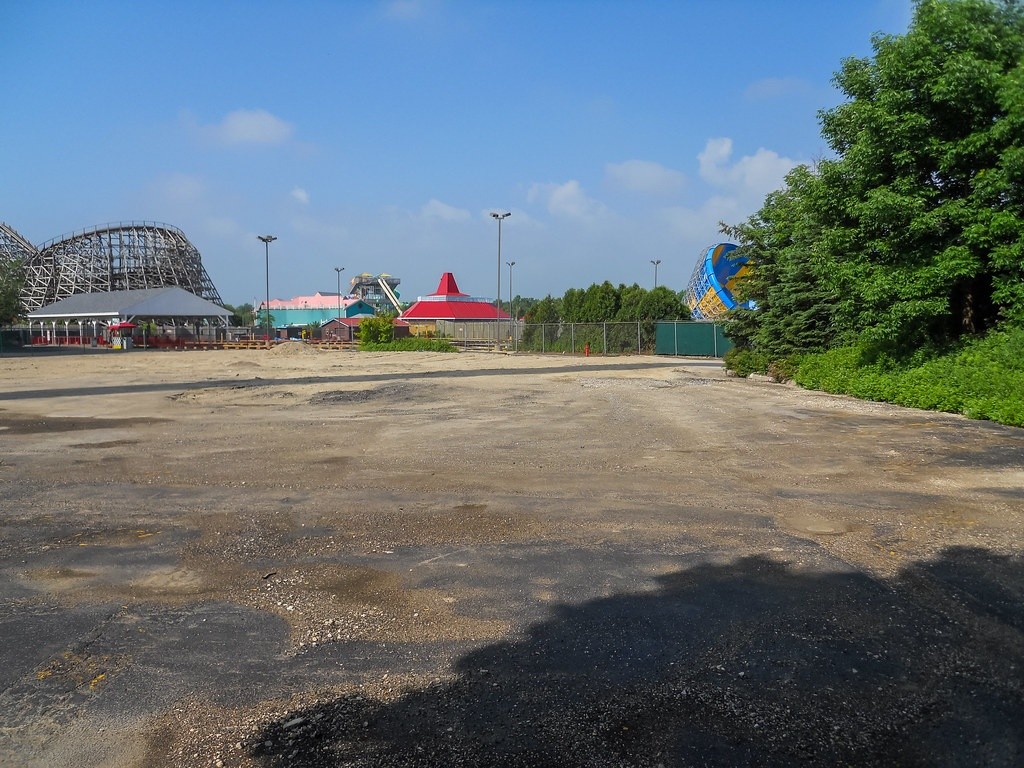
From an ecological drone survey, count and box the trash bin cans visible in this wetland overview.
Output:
[90,337,97,347]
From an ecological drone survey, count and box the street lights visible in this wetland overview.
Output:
[335,267,344,342]
[257,235,277,346]
[506,261,515,320]
[651,259,662,288]
[490,212,513,350]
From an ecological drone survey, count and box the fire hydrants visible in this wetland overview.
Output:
[585,342,591,357]
